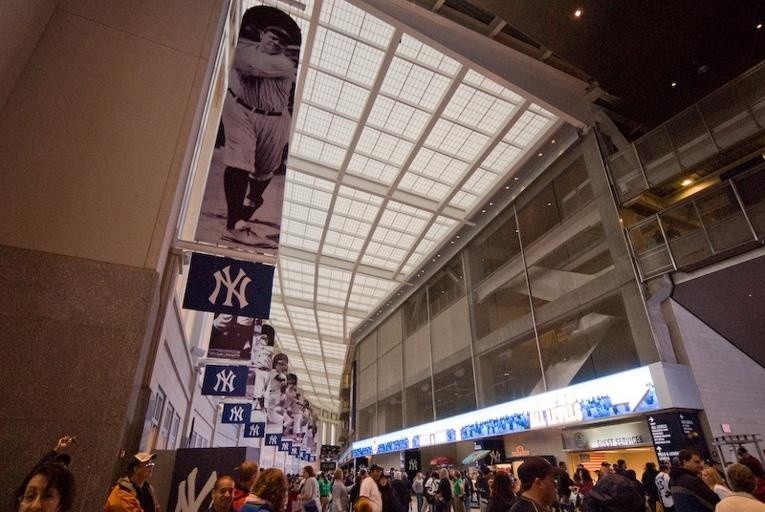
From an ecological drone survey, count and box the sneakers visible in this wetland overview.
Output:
[243,197,263,221]
[222,224,278,248]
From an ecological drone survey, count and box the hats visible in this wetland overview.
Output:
[579,473,645,512]
[128,453,156,467]
[517,456,563,479]
[264,26,291,43]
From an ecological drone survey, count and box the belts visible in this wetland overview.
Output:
[227,87,282,116]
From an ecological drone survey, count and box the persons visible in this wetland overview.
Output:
[16,438,78,511]
[105,453,159,511]
[642,446,765,510]
[413,469,473,511]
[508,455,647,512]
[210,461,412,511]
[475,469,517,511]
[215,312,312,437]
[221,26,292,231]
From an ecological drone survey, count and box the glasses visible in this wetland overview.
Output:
[138,461,155,468]
